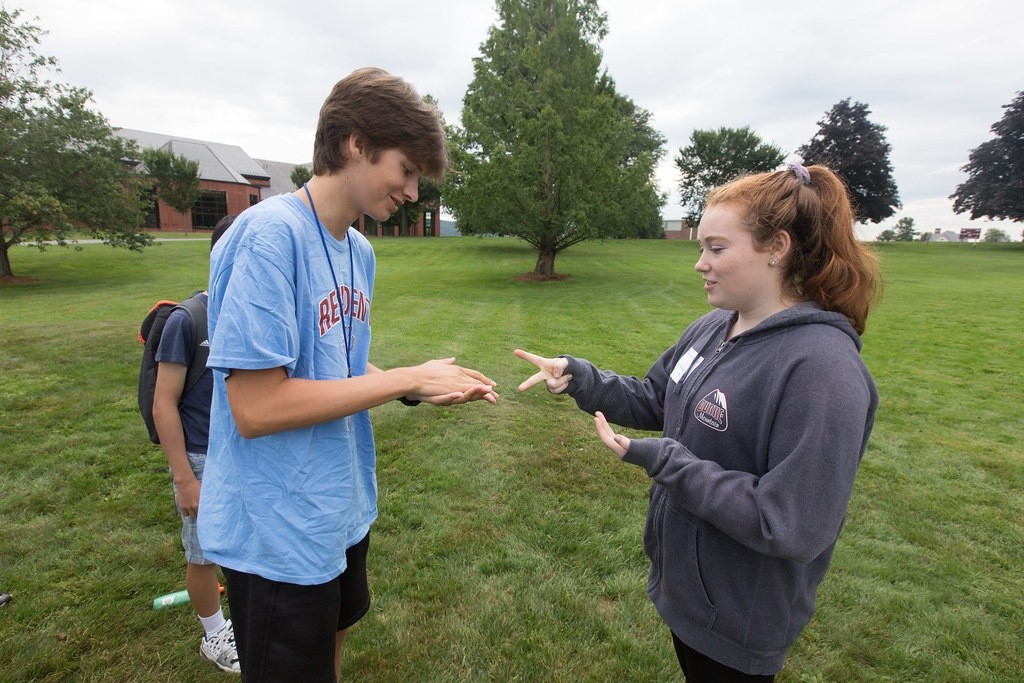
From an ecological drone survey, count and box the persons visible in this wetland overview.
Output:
[515,160,878,683]
[196,67,500,683]
[152,210,242,674]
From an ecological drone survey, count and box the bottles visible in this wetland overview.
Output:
[152,580,225,614]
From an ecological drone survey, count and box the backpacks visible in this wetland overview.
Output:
[136,291,210,445]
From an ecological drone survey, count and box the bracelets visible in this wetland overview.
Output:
[397,397,420,406]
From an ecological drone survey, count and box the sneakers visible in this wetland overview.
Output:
[198,618,242,673]
